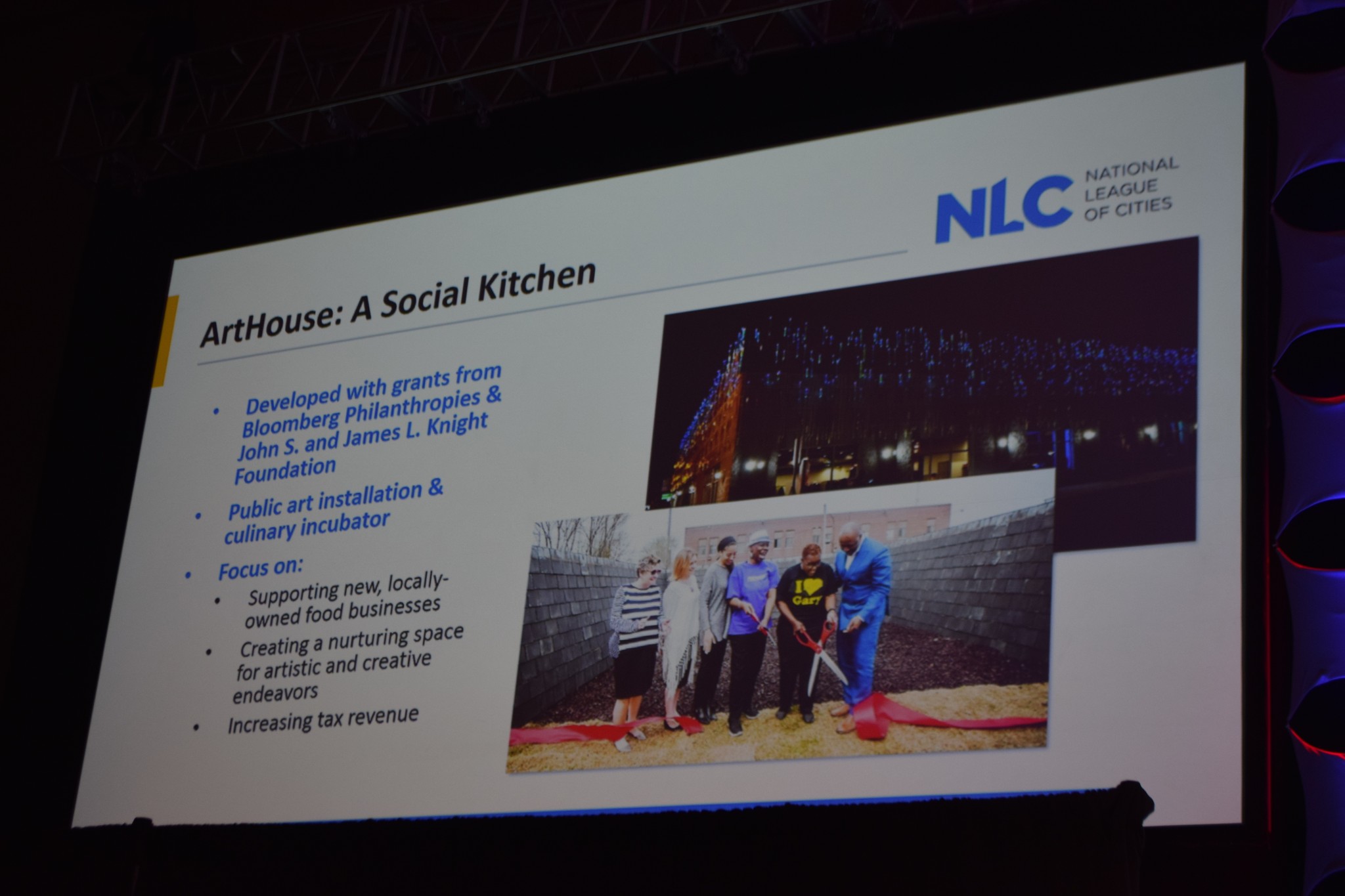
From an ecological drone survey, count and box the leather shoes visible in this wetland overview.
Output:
[803,712,814,723]
[831,704,850,717]
[837,715,857,734]
[776,708,787,720]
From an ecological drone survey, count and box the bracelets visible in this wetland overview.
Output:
[828,608,835,613]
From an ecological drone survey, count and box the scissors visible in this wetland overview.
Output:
[796,621,848,696]
[751,612,776,646]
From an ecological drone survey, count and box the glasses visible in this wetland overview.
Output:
[803,560,821,565]
[645,570,661,575]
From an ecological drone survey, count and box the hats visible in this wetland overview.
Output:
[717,536,735,552]
[747,531,772,547]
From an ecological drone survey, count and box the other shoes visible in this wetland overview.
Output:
[706,704,717,721]
[629,729,646,740]
[614,740,632,753]
[663,720,683,731]
[696,708,710,724]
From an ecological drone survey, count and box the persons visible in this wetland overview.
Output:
[727,530,780,737]
[691,538,737,725]
[775,543,838,724]
[829,522,892,733]
[608,554,662,752]
[661,549,700,732]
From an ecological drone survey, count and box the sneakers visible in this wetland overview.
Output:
[728,720,743,737]
[744,707,760,719]
